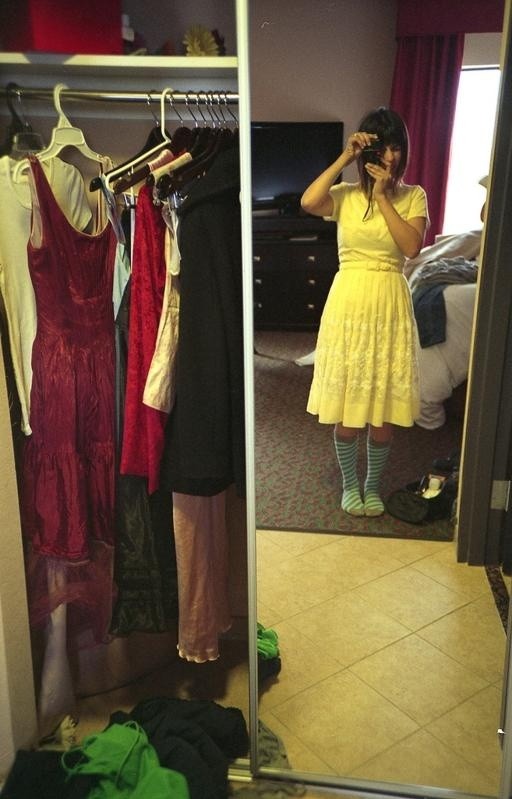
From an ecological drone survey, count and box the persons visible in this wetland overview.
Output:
[300,104,432,517]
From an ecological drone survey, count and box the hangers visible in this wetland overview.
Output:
[0,82,240,205]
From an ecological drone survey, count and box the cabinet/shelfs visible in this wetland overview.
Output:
[254,224,339,332]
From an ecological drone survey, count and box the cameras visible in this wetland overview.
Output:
[363,138,381,165]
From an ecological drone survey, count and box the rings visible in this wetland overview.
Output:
[352,132,358,138]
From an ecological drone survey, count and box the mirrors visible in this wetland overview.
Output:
[238,1,512,799]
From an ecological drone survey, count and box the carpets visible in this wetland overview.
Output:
[253,332,466,541]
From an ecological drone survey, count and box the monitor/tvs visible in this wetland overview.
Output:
[251,122,344,219]
